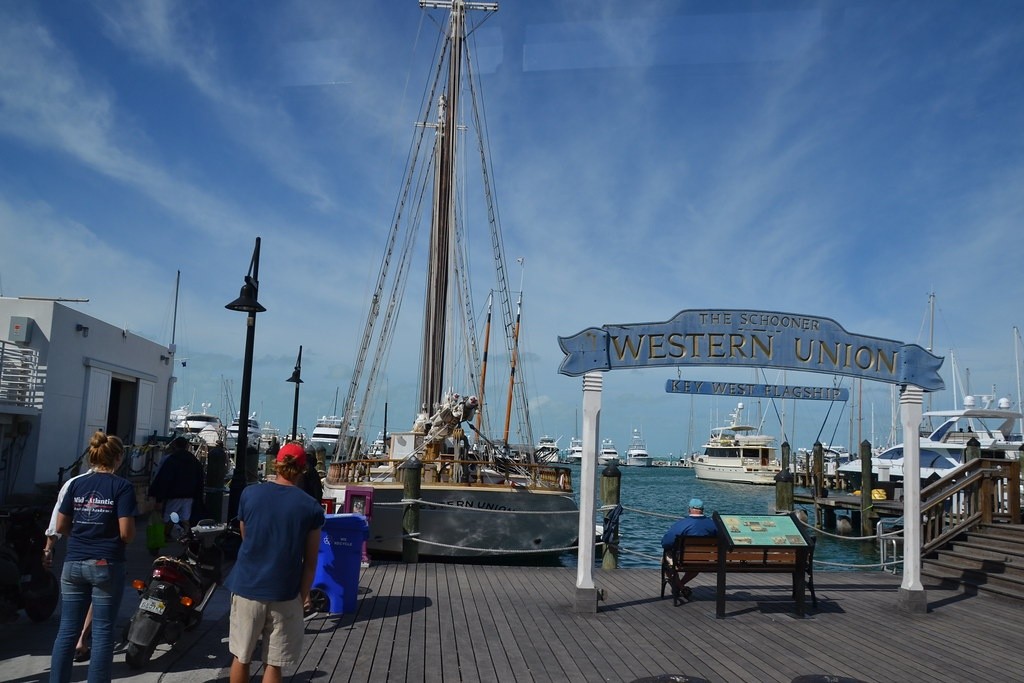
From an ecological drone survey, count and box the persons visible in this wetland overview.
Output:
[296,454,323,505]
[39,430,139,683]
[305,444,316,454]
[660,499,718,599]
[222,442,327,683]
[203,440,231,521]
[317,443,326,463]
[265,437,280,458]
[146,436,208,543]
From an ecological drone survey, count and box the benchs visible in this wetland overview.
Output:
[659,535,821,610]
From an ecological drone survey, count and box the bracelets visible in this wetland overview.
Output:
[42,548,55,552]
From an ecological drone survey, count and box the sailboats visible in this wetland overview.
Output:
[683,283,1024,558]
[318,1,608,566]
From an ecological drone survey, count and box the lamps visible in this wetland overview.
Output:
[76,323,89,337]
[168,344,176,357]
[159,355,171,365]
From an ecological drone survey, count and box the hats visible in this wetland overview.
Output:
[306,444,316,455]
[276,444,307,467]
[689,498,704,509]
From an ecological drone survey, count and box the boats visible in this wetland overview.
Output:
[534,433,564,463]
[597,438,621,466]
[625,421,654,467]
[169,377,394,466]
[565,435,583,465]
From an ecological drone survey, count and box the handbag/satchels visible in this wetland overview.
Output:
[145,505,166,549]
[27,569,59,624]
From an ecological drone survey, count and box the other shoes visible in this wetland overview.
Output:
[303,607,319,621]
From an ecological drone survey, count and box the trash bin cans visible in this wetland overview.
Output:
[309,513,368,614]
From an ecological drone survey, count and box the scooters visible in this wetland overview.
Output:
[125,510,241,669]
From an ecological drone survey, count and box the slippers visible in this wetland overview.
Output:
[73,647,91,660]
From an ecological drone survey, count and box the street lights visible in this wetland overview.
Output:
[286,344,304,441]
[224,237,266,558]
